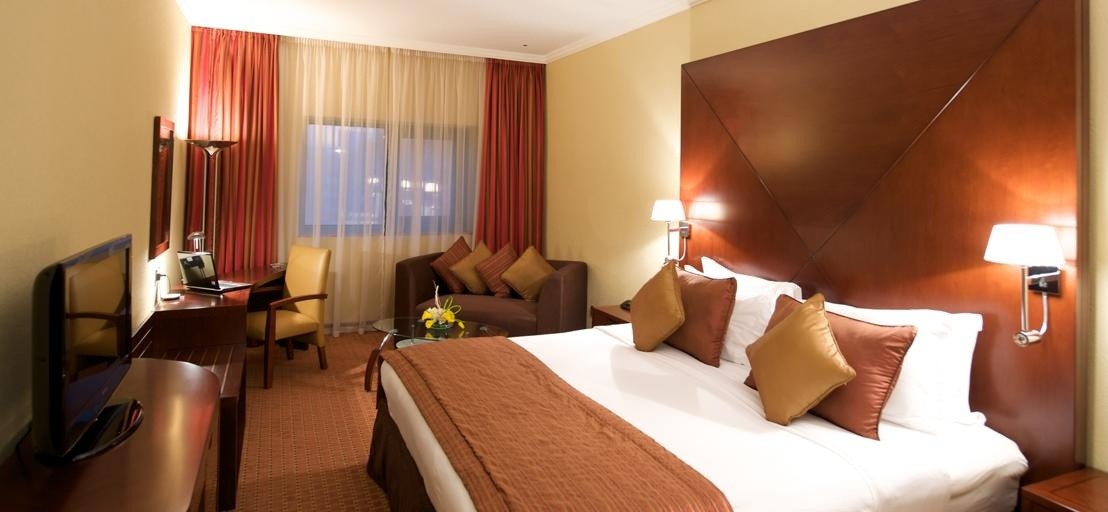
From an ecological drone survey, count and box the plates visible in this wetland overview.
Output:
[161,294,180,298]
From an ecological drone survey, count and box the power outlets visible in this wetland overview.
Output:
[155,266,161,282]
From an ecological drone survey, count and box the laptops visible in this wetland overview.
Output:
[175,249,253,294]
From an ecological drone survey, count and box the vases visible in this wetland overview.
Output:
[427,321,453,329]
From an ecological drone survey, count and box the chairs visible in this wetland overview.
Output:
[247,245,332,390]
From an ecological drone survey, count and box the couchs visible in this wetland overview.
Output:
[395,251,588,336]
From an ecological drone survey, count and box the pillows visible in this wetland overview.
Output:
[431,236,473,294]
[663,265,737,368]
[450,240,494,295]
[630,264,684,351]
[700,256,801,367]
[502,247,559,304]
[745,292,856,427]
[475,244,519,298]
[743,295,917,440]
[826,298,986,442]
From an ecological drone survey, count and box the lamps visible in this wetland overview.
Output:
[983,224,1065,349]
[184,139,239,265]
[652,200,692,263]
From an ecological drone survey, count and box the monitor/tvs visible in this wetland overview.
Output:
[31,233,144,470]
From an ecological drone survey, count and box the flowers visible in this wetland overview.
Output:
[421,281,466,329]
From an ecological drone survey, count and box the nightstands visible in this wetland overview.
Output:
[1020,469,1108,511]
[591,305,632,326]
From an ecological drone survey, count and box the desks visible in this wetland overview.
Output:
[1,359,220,511]
[152,262,287,357]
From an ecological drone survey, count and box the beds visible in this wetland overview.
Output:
[366,322,1030,510]
